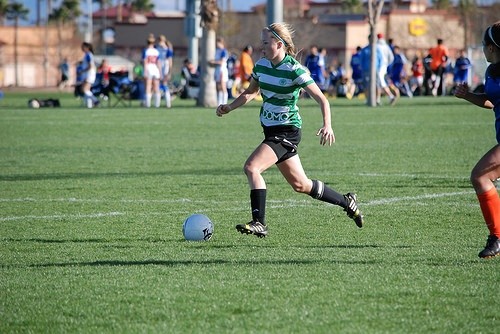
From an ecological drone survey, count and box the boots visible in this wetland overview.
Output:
[154,94,161,107]
[143,94,151,107]
[163,94,171,107]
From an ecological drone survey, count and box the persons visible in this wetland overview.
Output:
[179,34,472,105]
[454,21,500,259]
[217,22,364,238]
[59,42,110,108]
[141,33,173,109]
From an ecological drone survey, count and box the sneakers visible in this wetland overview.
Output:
[343,193,363,228]
[93,101,100,108]
[478,235,500,258]
[236,220,267,237]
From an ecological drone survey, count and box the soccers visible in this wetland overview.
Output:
[180,214,215,242]
[30,100,41,109]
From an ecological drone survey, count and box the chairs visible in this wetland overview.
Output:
[75,72,139,107]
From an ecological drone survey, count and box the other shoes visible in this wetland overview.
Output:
[390,98,396,105]
[346,93,352,99]
[358,94,366,100]
[432,89,437,95]
